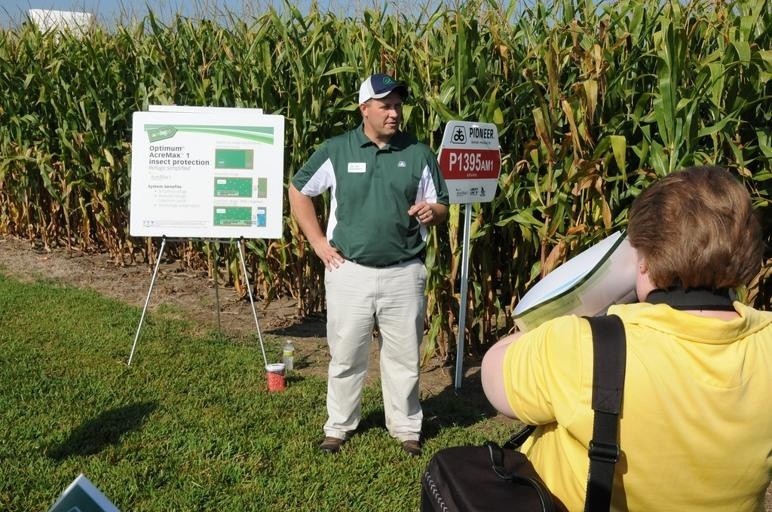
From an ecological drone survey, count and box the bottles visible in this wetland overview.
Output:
[282,339,295,371]
[265,362,286,393]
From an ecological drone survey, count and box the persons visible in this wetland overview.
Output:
[478,164,772,512]
[288,73,450,455]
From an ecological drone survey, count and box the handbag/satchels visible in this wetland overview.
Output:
[421,440,555,512]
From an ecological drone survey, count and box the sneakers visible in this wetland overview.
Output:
[402,440,423,456]
[319,437,346,453]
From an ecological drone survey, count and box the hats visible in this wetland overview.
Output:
[358,73,408,106]
